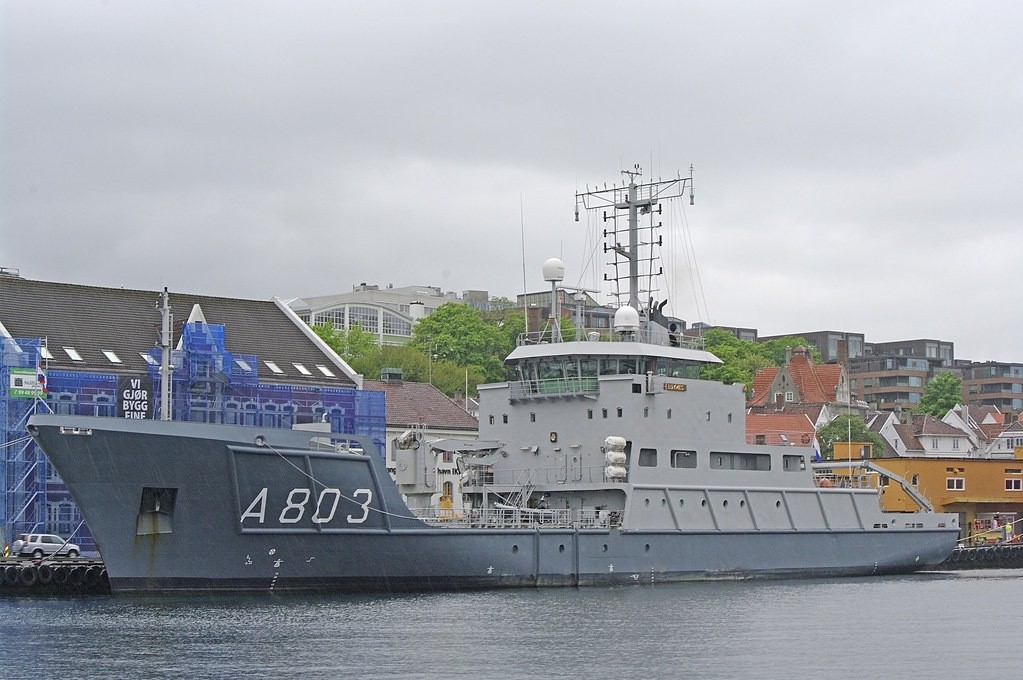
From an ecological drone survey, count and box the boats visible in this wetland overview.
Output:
[26,163,961,596]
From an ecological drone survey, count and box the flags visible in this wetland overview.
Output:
[814,439,821,462]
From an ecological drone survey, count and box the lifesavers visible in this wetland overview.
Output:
[0,565,105,587]
[945,547,1023,563]
[801,434,810,444]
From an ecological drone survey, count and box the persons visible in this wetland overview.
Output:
[1005,523,1012,541]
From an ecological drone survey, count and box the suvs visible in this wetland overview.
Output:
[12,533,81,557]
